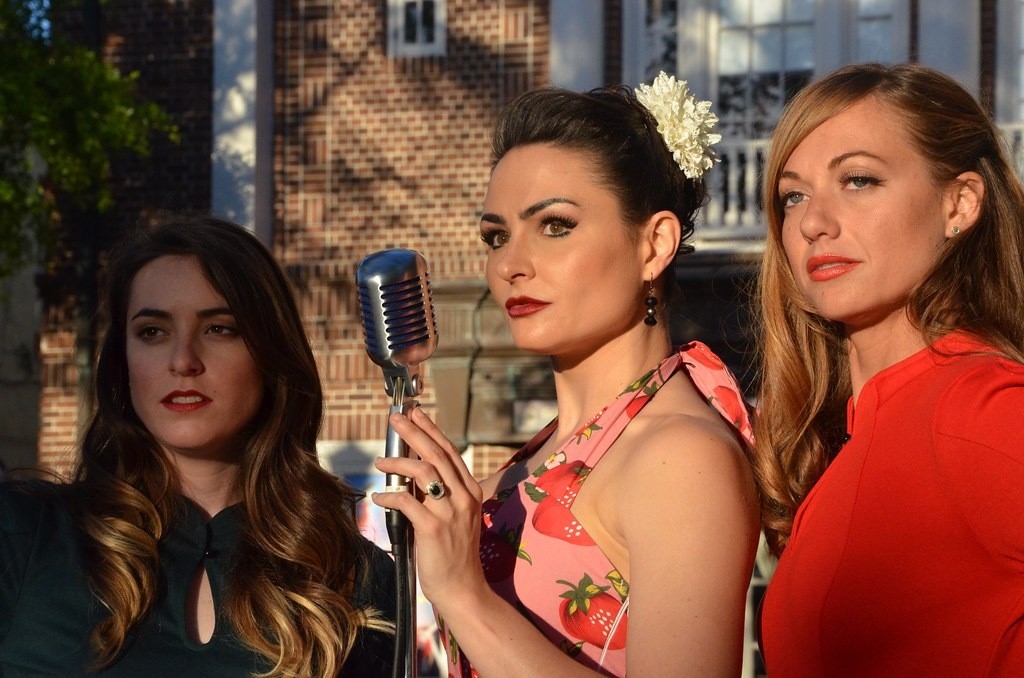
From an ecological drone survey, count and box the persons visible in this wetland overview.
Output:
[759,66,1024,678]
[0,212,400,678]
[365,68,764,678]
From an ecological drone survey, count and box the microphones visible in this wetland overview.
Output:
[356,247,441,558]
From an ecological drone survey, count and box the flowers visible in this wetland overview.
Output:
[636,71,723,183]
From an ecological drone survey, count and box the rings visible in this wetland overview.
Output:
[427,480,448,499]
